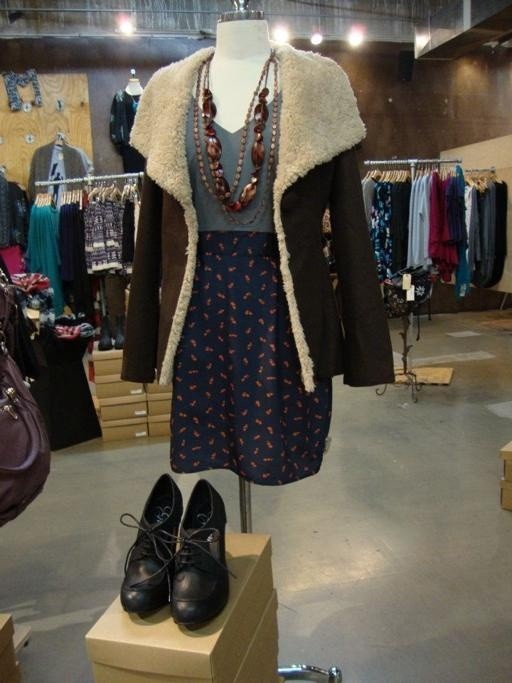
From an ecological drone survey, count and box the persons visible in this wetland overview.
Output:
[109,79,146,174]
[120,10,397,487]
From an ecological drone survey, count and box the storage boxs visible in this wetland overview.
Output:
[499,478,512,510]
[499,441,512,480]
[0,613,22,683]
[84,535,282,683]
[91,349,173,442]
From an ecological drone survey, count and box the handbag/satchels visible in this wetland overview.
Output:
[1,348,54,527]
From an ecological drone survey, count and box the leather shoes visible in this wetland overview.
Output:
[119,474,184,614]
[172,476,228,626]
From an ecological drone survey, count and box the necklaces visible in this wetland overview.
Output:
[192,52,278,226]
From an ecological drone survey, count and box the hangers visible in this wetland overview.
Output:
[365,161,503,193]
[33,172,142,211]
